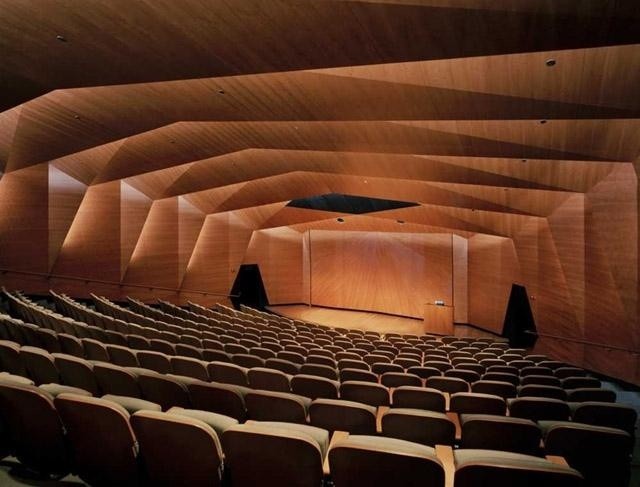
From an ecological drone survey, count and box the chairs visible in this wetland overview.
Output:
[0,287,639,486]
